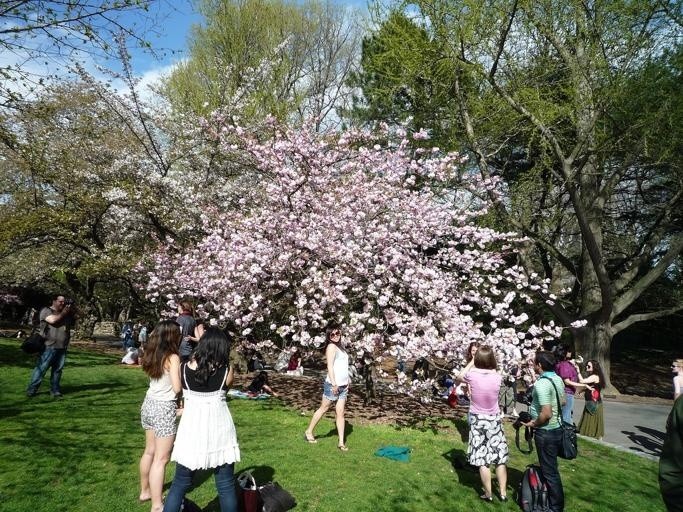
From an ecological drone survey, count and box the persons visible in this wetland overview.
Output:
[117,298,303,512]
[521,351,570,512]
[574,359,605,441]
[305,324,350,451]
[458,342,519,502]
[25,295,72,397]
[658,392,683,512]
[671,359,683,405]
[29,308,40,336]
[554,343,595,432]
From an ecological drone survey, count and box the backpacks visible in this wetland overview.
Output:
[515,466,549,512]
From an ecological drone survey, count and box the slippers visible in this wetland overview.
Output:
[337,446,347,450]
[302,437,315,441]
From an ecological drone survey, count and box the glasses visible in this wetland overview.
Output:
[330,331,339,338]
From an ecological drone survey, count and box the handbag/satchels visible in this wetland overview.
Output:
[557,429,576,460]
[21,333,44,354]
[234,477,294,512]
[374,447,410,461]
[448,394,458,408]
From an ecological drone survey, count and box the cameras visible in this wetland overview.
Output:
[512,410,532,431]
[64,298,75,304]
